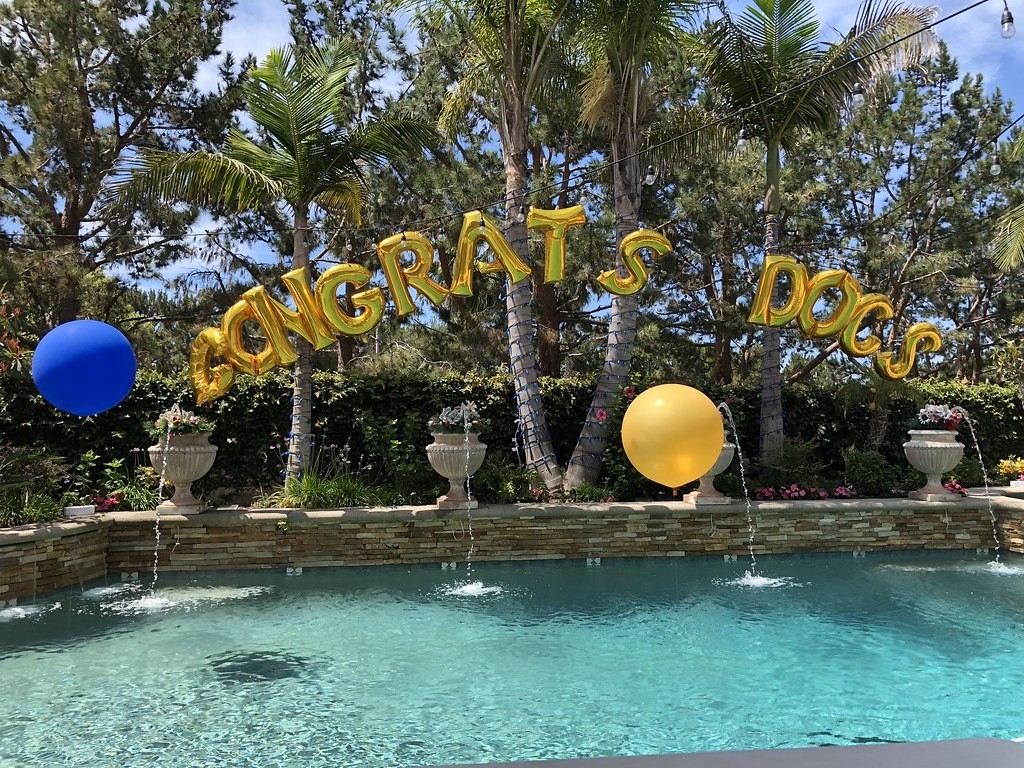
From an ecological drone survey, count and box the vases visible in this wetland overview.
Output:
[902,430,965,502]
[147,433,218,515]
[425,432,487,510]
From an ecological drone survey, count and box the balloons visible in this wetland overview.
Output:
[620,383,725,496]
[31,321,136,416]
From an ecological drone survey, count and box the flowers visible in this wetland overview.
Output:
[908,403,979,432]
[143,409,218,442]
[427,400,492,435]
